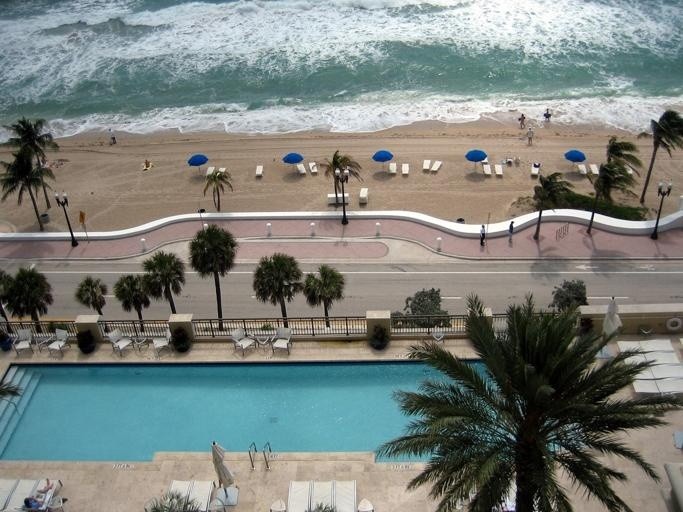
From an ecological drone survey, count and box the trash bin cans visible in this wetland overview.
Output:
[198,209,206,213]
[40,214,50,224]
[456,217,465,223]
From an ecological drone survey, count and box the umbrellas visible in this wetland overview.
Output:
[187,154,209,173]
[282,152,304,172]
[209,440,235,499]
[372,149,393,173]
[465,149,487,168]
[565,149,586,169]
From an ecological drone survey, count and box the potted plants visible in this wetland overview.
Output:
[76,331,95,352]
[369,324,388,349]
[172,327,189,353]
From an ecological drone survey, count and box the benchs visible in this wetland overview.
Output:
[359,188,368,204]
[326,193,349,204]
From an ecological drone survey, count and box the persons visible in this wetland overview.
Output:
[518,114,526,130]
[527,128,535,146]
[143,159,152,168]
[545,108,552,122]
[23,478,53,508]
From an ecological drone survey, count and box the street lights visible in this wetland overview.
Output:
[334,167,350,225]
[54,188,80,248]
[650,182,672,241]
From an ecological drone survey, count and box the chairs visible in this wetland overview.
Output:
[9,328,70,356]
[287,479,356,512]
[162,479,214,512]
[255,165,263,176]
[106,328,172,357]
[205,166,227,179]
[388,162,409,175]
[531,163,539,176]
[579,162,600,178]
[231,327,293,353]
[0,479,63,512]
[481,156,502,175]
[296,161,318,174]
[422,159,442,172]
